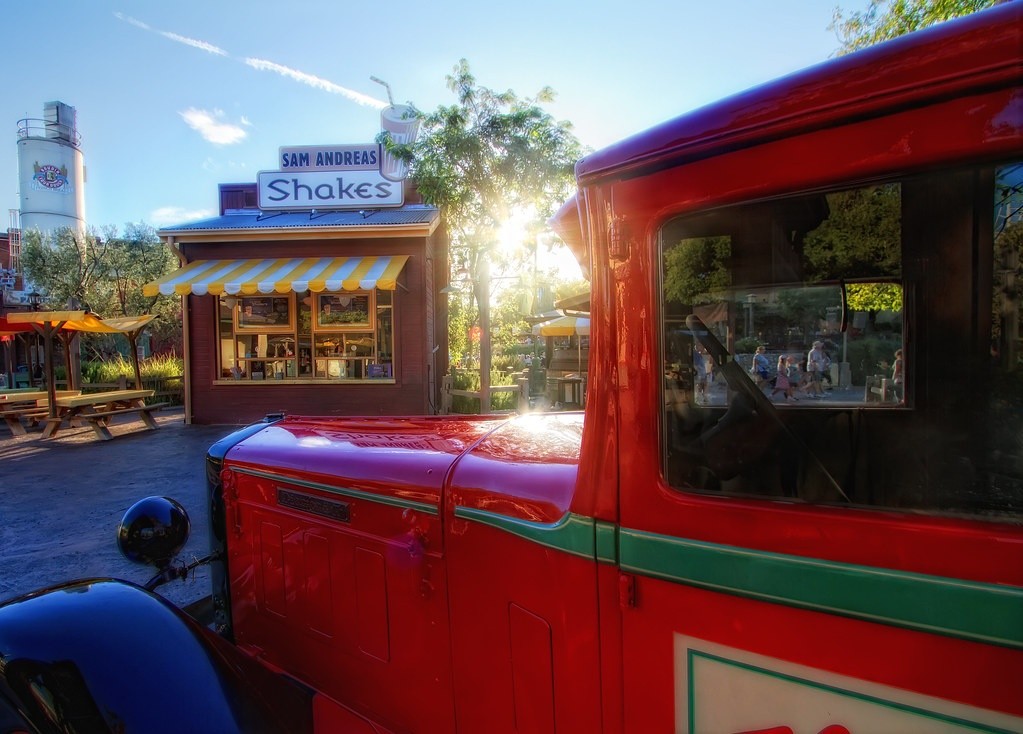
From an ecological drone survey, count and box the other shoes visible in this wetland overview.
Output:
[803,393,832,400]
[767,394,776,403]
[704,399,708,402]
[787,396,799,402]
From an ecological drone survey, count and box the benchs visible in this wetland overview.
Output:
[0,403,172,419]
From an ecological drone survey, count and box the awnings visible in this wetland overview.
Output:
[142,255,412,296]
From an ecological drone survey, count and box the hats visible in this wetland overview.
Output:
[779,355,787,359]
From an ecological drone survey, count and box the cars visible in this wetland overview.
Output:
[117,0,1023,734]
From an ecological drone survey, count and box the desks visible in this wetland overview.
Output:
[0,390,82,436]
[37,389,160,440]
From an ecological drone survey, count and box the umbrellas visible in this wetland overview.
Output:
[532,317,590,377]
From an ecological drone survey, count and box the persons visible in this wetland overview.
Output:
[891,349,904,404]
[693,343,708,404]
[752,340,832,402]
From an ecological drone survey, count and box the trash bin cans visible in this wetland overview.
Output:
[547,377,583,408]
[534,368,546,392]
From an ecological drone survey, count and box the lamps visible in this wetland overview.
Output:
[256,212,263,221]
[310,209,317,219]
[359,209,366,219]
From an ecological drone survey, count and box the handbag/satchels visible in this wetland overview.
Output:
[762,371,769,379]
